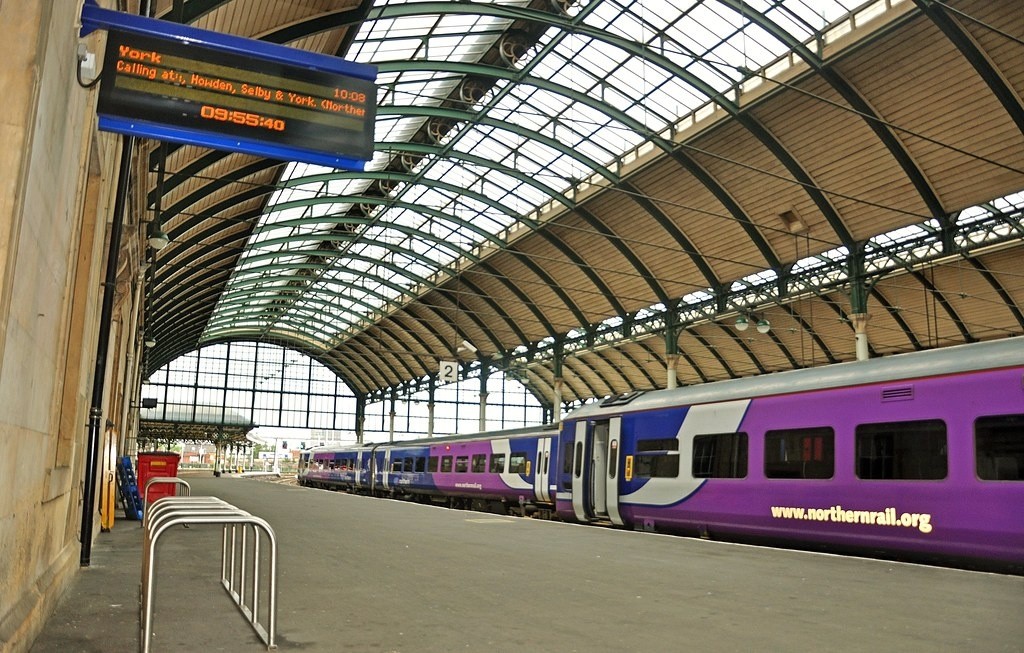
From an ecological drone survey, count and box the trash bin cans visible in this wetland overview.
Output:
[136,450,181,504]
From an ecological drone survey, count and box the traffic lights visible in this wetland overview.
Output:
[301,442,305,449]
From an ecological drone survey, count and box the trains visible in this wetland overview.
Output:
[295,333,1024,578]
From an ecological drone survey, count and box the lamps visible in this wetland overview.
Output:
[142,374,150,384]
[402,394,419,405]
[734,304,770,333]
[140,218,170,250]
[505,363,530,384]
[138,331,156,347]
